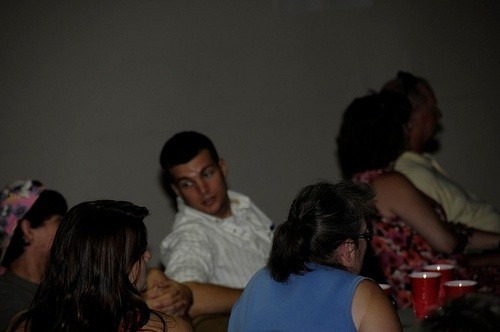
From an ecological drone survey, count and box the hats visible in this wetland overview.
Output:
[0,180,46,264]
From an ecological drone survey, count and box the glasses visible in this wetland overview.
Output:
[335,229,372,245]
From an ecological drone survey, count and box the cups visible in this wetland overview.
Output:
[378,284,391,298]
[398,263,478,320]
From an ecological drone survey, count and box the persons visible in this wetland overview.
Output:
[382,71,500,233]
[335,92,500,311]
[159,131,277,317]
[0,179,500,332]
